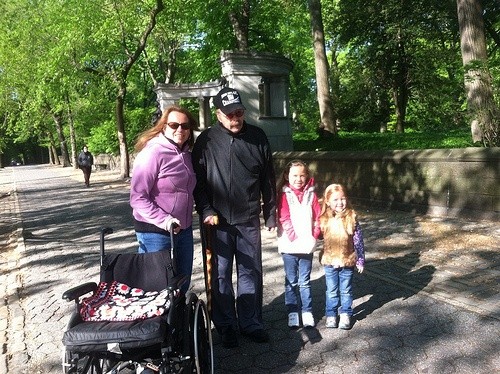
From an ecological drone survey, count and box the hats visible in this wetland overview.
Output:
[213,87,247,115]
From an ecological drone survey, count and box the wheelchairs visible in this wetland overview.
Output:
[61,222,214,374]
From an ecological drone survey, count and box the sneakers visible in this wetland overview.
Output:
[288,312,299,327]
[338,313,353,328]
[301,311,315,328]
[325,317,339,327]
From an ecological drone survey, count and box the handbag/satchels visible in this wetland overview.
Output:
[78,153,82,169]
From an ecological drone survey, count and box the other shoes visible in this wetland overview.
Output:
[221,330,239,348]
[240,328,269,343]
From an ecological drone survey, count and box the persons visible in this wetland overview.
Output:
[276,159,322,328]
[313,183,365,329]
[78,146,93,187]
[190,88,277,341]
[130,107,197,296]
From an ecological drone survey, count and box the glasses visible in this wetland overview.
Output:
[215,107,244,119]
[164,122,190,130]
